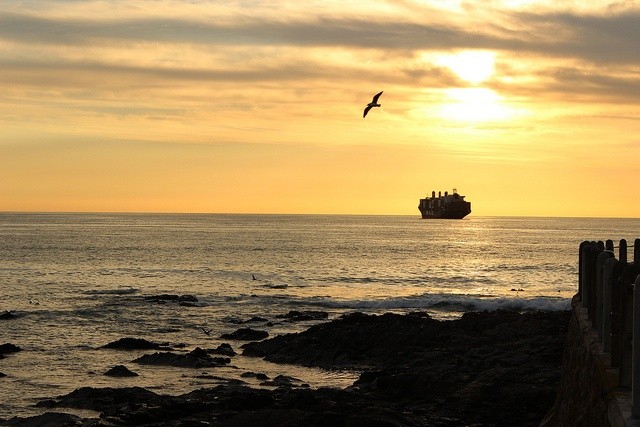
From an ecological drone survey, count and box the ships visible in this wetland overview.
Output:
[418,189,471,219]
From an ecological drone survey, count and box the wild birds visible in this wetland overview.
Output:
[363,91,382,118]
